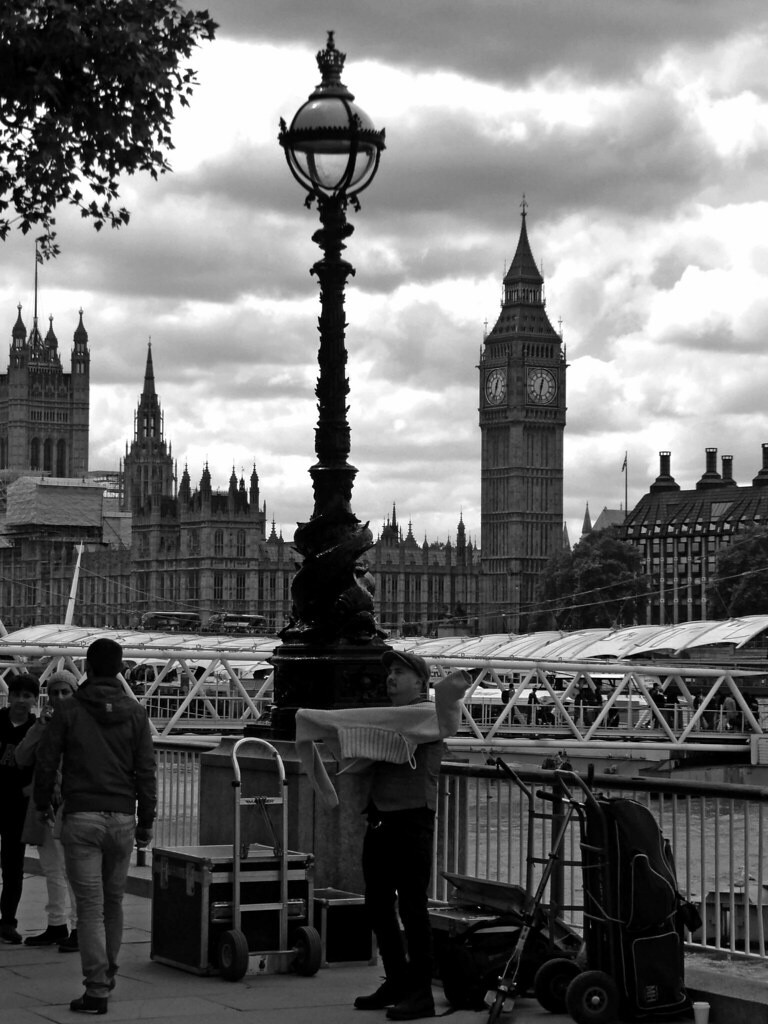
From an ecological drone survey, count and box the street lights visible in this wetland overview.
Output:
[264,30,395,713]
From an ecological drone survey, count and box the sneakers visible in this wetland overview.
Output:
[69,992,107,1015]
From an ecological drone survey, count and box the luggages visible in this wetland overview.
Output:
[534,769,700,1024]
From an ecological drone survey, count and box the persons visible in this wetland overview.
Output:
[502,683,760,733]
[296,649,473,1021]
[0,669,81,953]
[32,638,158,1014]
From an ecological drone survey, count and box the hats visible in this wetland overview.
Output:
[47,670,78,695]
[381,650,431,684]
[8,672,39,698]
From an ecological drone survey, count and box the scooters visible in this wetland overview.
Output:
[483,787,592,1024]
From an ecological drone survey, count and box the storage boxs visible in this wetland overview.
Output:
[149,843,315,978]
[313,887,378,969]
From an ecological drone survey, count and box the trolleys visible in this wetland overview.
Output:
[491,753,629,1024]
[147,736,326,982]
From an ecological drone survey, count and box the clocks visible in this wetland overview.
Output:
[485,368,506,405]
[527,368,557,405]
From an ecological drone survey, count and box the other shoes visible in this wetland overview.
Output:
[354,976,411,1010]
[59,930,79,950]
[386,986,435,1019]
[24,925,68,946]
[0,924,22,944]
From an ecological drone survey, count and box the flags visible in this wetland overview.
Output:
[621,456,627,472]
